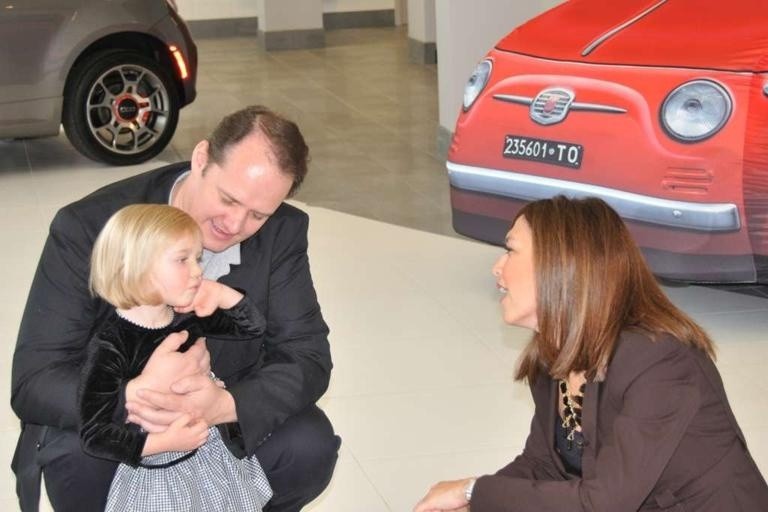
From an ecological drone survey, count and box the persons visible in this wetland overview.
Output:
[9,104,342,512]
[410,194,768,512]
[75,203,274,511]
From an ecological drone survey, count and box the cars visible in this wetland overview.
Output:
[446,2,767,282]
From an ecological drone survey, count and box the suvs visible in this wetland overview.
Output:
[0,1,200,165]
